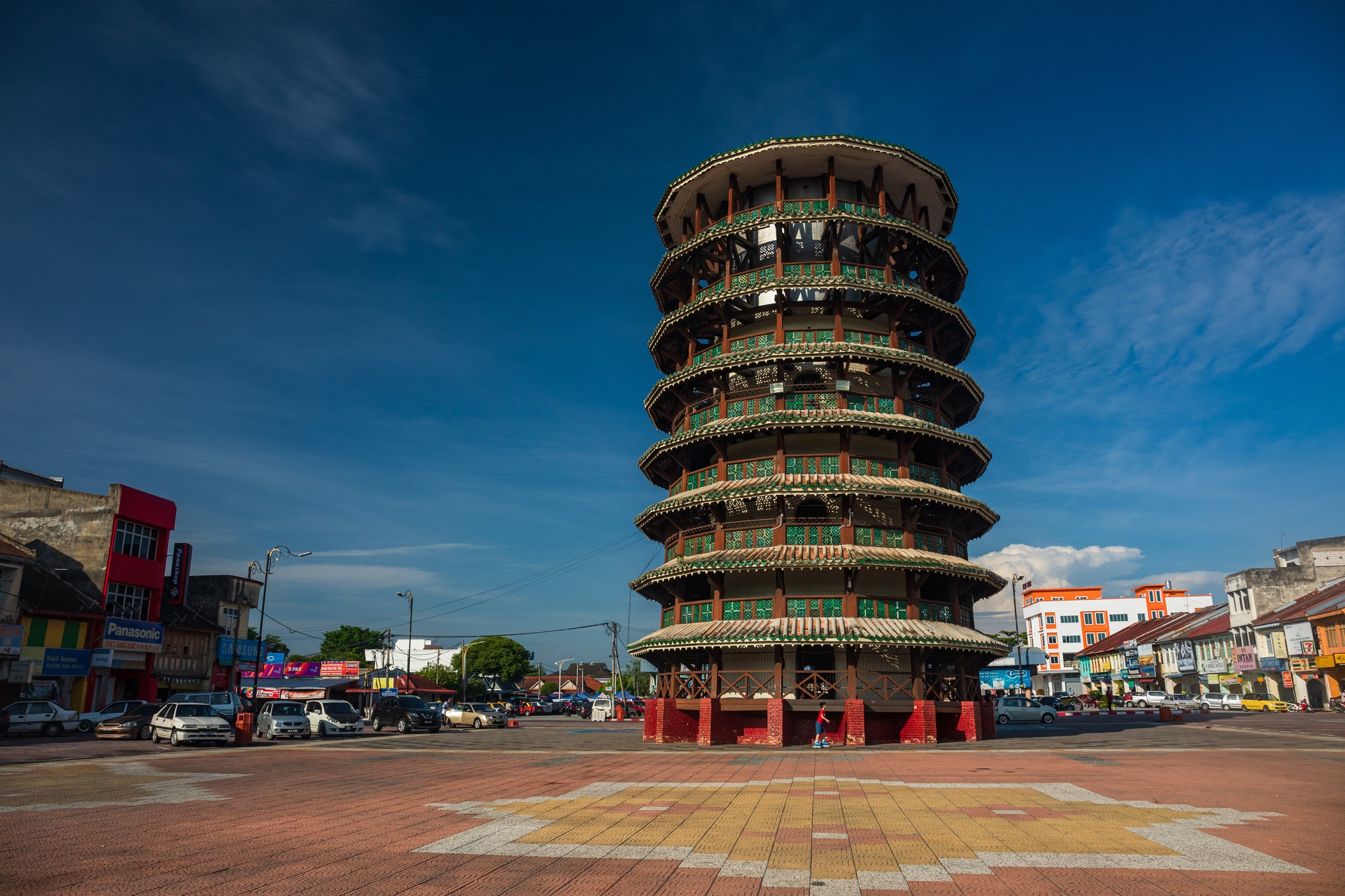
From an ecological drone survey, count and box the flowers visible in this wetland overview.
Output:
[1061,696,1083,706]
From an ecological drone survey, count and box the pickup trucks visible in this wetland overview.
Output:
[1131,691,1172,708]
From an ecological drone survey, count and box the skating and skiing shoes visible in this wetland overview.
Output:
[820,738,829,747]
[812,740,821,748]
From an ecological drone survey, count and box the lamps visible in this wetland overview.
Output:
[1188,594,1190,595]
[1166,594,1168,596]
[1079,597,1081,599]
[1100,597,1102,598]
[835,379,851,391]
[1142,595,1144,597]
[1058,598,1060,600]
[1209,593,1211,595]
[1027,603,1029,605]
[1032,601,1034,603]
[1022,605,1024,607]
[770,381,786,393]
[1038,599,1040,600]
[1121,596,1122,598]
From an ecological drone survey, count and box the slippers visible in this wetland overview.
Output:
[442,726,448,728]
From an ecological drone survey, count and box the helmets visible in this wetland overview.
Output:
[508,701,510,702]
[568,700,571,702]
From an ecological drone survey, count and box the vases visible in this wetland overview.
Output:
[1066,705,1074,711]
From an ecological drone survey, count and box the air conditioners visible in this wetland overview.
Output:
[1072,664,1078,669]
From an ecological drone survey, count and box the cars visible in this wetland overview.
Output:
[1111,695,1124,707]
[1220,694,1248,711]
[239,695,645,741]
[1200,692,1226,710]
[1002,691,1084,711]
[1159,693,1200,710]
[77,700,231,747]
[1329,695,1341,710]
[992,696,1058,725]
[1078,694,1089,707]
[1241,693,1288,713]
[1175,693,1201,704]
[1085,693,1104,708]
[0,701,80,740]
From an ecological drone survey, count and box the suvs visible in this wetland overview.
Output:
[1123,692,1144,707]
[166,691,244,722]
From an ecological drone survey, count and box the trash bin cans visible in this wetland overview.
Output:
[1160,707,1172,721]
[234,713,254,745]
[615,705,624,718]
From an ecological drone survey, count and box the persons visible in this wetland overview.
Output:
[564,700,573,715]
[1106,687,1115,712]
[1341,690,1345,705]
[814,702,830,746]
[442,698,451,728]
[506,701,515,716]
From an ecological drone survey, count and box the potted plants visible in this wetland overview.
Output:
[1089,690,1116,711]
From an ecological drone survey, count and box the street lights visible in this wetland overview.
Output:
[458,641,486,703]
[611,668,628,695]
[555,658,576,702]
[396,590,413,696]
[1012,574,1027,707]
[237,545,313,715]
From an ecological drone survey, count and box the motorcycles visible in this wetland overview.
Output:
[1286,696,1315,713]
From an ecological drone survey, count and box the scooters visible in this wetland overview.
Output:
[1332,700,1345,713]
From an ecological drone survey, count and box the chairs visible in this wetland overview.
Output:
[195,708,204,716]
[287,707,294,714]
